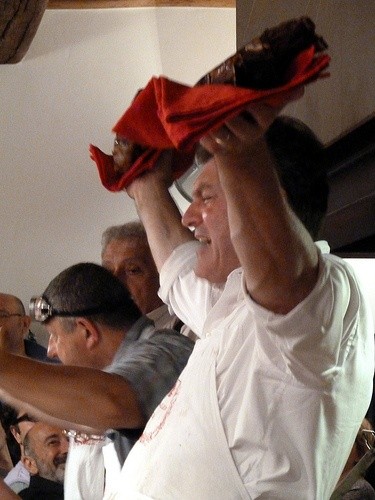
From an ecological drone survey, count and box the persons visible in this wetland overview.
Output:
[332,418,375,500]
[0,262,196,500]
[0,420,70,500]
[3,410,37,494]
[0,293,60,437]
[103,116,375,500]
[98,222,196,341]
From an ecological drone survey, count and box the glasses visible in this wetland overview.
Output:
[0,308,24,324]
[29,296,110,323]
[11,412,40,426]
